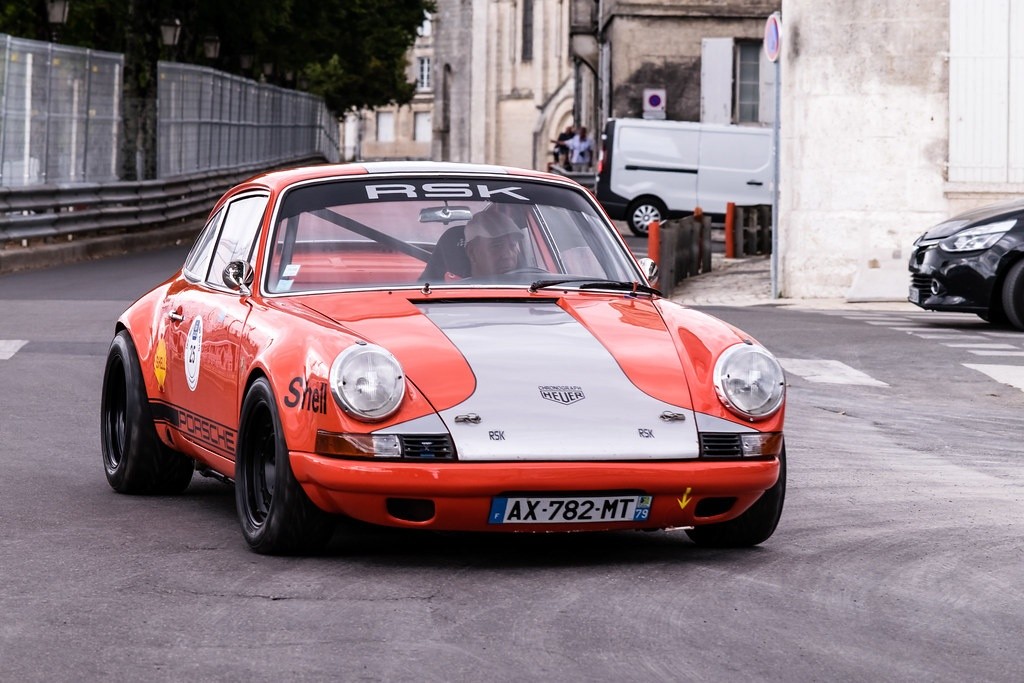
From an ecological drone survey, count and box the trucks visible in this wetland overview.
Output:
[595,117,775,238]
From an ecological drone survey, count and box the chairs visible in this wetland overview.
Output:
[417,225,470,285]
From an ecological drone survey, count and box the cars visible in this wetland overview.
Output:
[906,198,1024,332]
[100,161,789,559]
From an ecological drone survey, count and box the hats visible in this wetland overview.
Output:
[464,211,524,248]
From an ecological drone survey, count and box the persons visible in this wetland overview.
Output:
[549,127,593,173]
[457,210,525,285]
